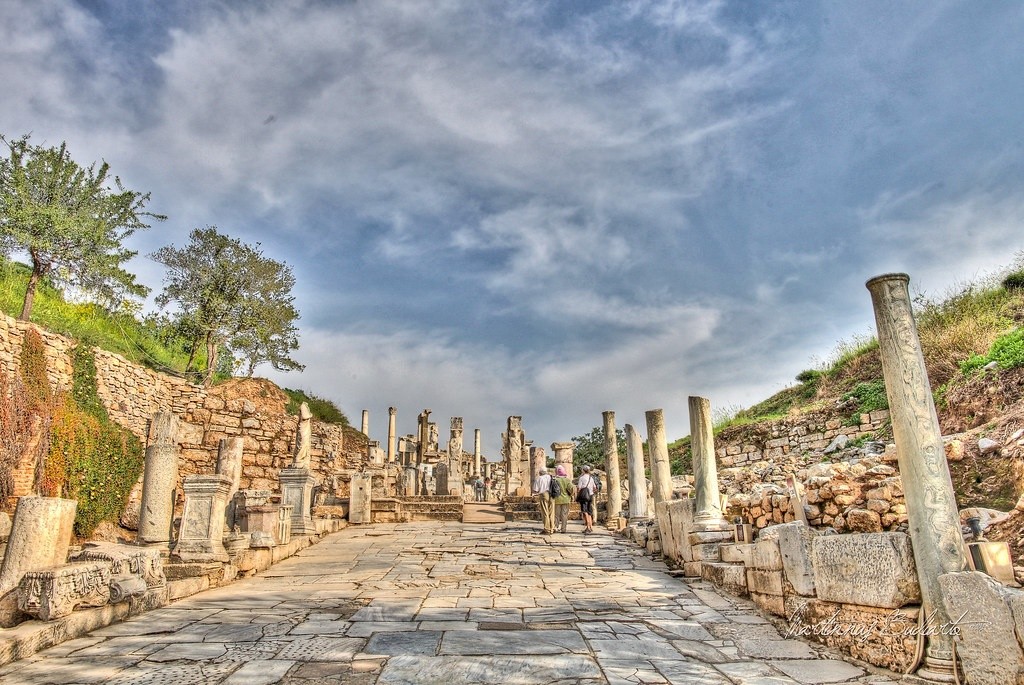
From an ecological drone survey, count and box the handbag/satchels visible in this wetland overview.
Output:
[550,480,561,498]
[575,487,591,502]
[565,485,574,495]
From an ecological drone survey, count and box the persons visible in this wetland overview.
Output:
[578,466,596,534]
[474,476,491,502]
[533,466,555,534]
[450,430,462,457]
[509,429,520,454]
[554,465,571,533]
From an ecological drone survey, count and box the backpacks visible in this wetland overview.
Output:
[477,483,484,488]
[594,477,603,492]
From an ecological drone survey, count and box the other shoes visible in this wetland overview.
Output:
[582,529,591,533]
[539,530,550,535]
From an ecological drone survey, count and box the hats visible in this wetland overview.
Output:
[582,465,590,473]
[538,466,548,474]
